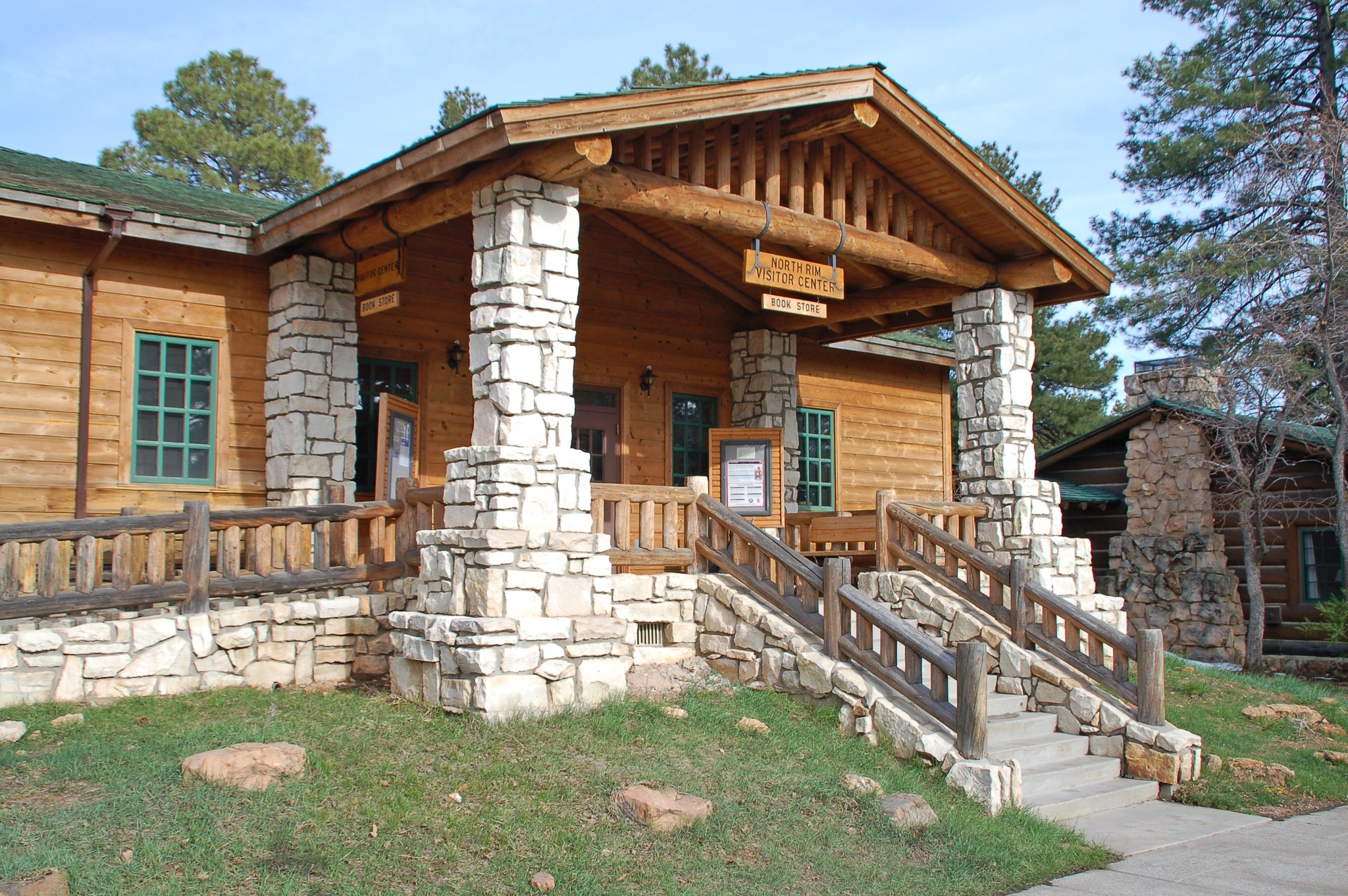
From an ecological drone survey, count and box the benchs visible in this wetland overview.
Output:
[798,515,877,556]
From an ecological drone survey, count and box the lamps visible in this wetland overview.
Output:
[446,340,467,376]
[640,365,657,398]
